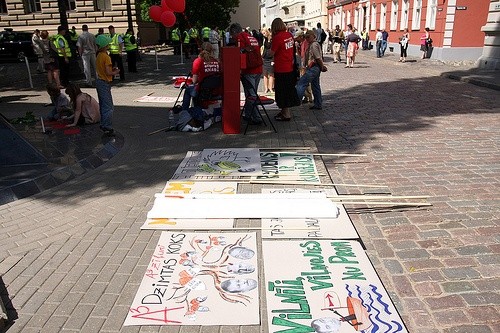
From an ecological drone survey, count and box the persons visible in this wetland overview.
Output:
[380,29,389,56]
[269,18,301,121]
[229,23,264,125]
[398,28,411,63]
[420,27,430,59]
[31,25,146,89]
[361,28,369,50]
[169,18,361,125]
[61,85,101,128]
[94,34,120,131]
[375,28,383,59]
[44,83,73,120]
[297,30,328,110]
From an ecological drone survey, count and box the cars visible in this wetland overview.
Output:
[0,31,36,64]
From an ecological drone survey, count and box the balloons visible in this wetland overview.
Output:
[149,0,186,27]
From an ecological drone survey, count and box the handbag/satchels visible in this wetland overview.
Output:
[293,62,300,82]
[425,37,433,44]
[48,39,59,58]
[240,33,264,70]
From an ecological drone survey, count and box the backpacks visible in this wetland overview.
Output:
[320,29,327,42]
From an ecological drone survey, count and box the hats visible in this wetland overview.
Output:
[108,25,115,29]
[294,30,304,38]
[95,35,113,49]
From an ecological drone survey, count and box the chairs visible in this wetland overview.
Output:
[239,77,277,136]
[174,72,224,130]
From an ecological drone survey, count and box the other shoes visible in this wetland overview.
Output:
[402,60,406,63]
[274,112,291,121]
[242,115,265,123]
[57,85,64,89]
[350,66,353,68]
[309,106,322,110]
[333,61,337,63]
[102,128,114,132]
[344,65,349,68]
[399,59,402,62]
